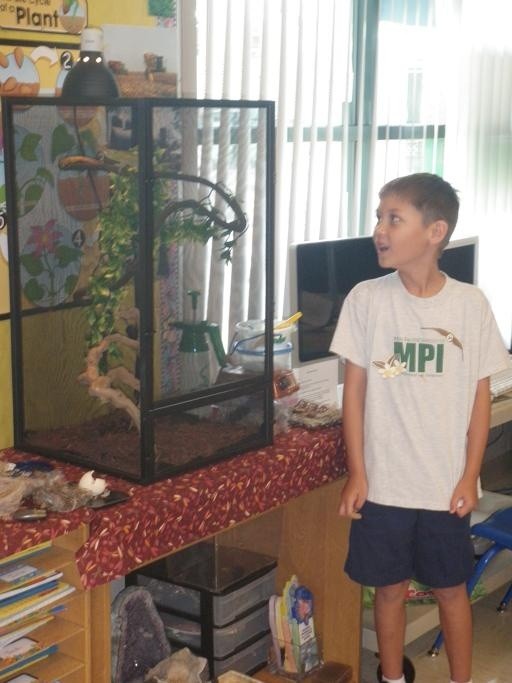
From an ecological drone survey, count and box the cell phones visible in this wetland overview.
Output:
[85,488,130,508]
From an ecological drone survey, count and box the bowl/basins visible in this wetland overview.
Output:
[235,343,293,376]
[235,313,296,351]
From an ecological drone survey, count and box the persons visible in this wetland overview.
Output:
[327,174,511,683]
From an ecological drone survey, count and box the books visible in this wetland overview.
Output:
[0,537,77,683]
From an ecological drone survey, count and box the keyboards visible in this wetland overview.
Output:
[490,353,511,398]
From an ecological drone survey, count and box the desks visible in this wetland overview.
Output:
[344,368,512,501]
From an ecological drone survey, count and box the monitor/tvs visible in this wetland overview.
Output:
[288,233,397,372]
[437,233,479,287]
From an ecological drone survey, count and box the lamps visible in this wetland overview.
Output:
[61,0,120,97]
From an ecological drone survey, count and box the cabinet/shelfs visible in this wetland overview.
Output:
[125,543,278,683]
[0,385,363,682]
[0,95,275,486]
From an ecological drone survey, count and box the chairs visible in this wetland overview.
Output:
[427,507,510,656]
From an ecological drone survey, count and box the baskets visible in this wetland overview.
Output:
[268,632,322,679]
